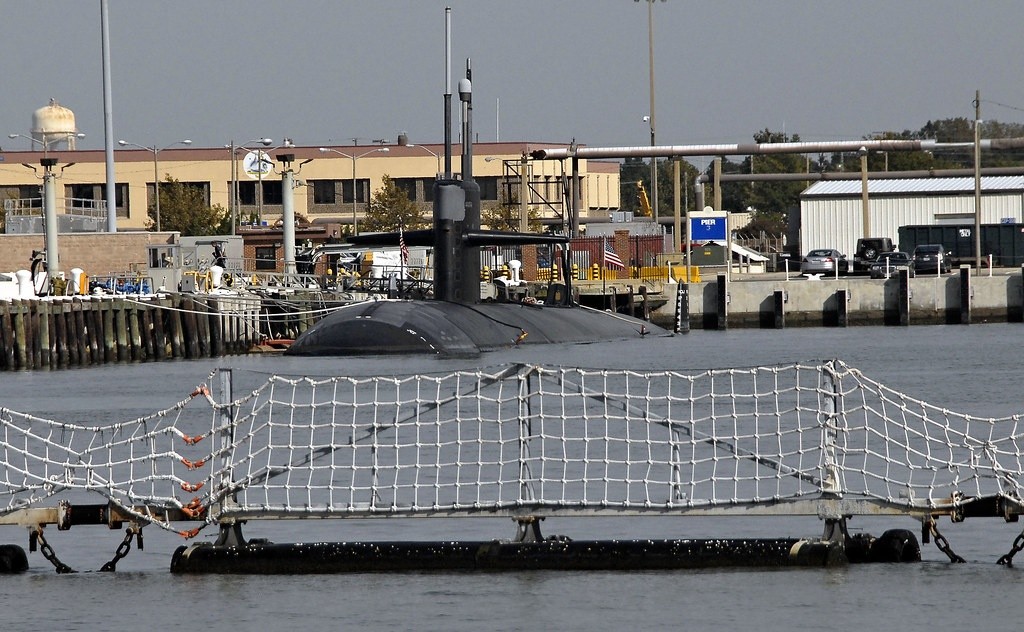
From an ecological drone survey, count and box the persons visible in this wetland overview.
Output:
[210,241,225,268]
[154,253,169,267]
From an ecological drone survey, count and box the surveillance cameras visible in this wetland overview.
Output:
[643,116,650,123]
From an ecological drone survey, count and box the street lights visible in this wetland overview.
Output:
[6,132,81,264]
[115,132,440,277]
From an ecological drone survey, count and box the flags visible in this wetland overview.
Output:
[604,239,624,267]
[399,228,408,264]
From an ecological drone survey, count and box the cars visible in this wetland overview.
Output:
[852,237,896,272]
[871,251,916,278]
[912,244,953,274]
[800,249,848,275]
